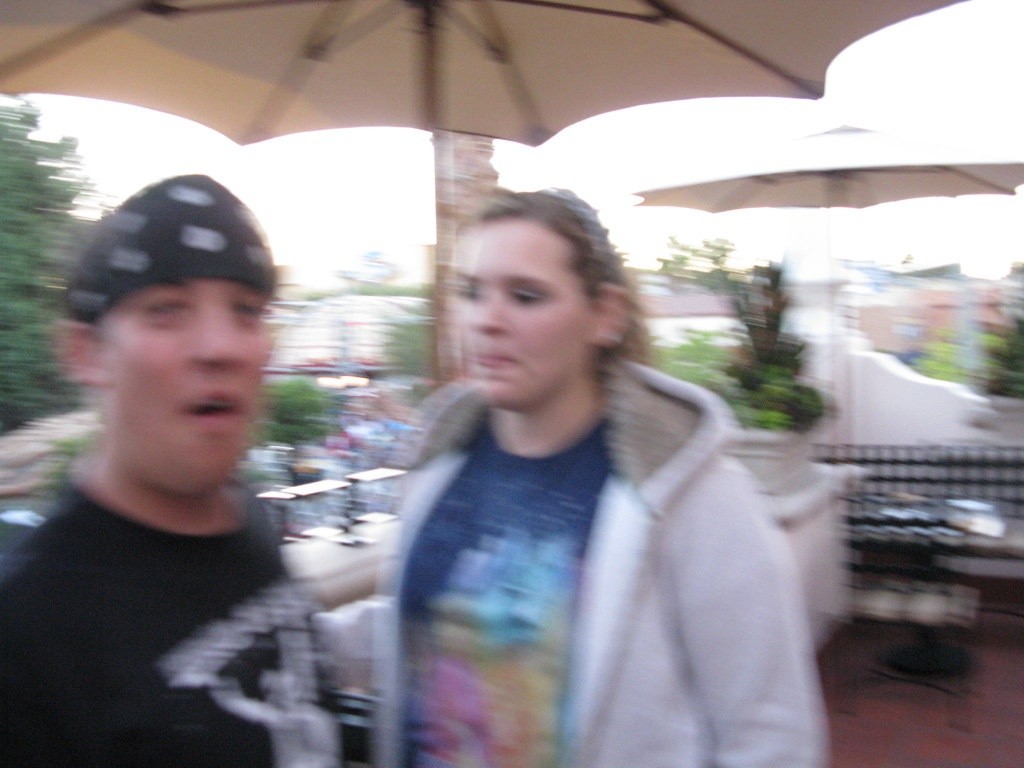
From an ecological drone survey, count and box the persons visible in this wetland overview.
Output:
[0,175,347,768]
[375,193,829,768]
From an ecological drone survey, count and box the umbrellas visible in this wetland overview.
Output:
[633,122,1024,209]
[1,0,974,381]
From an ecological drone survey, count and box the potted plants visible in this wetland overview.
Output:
[714,267,824,494]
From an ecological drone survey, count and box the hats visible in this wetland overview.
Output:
[66,175,273,323]
